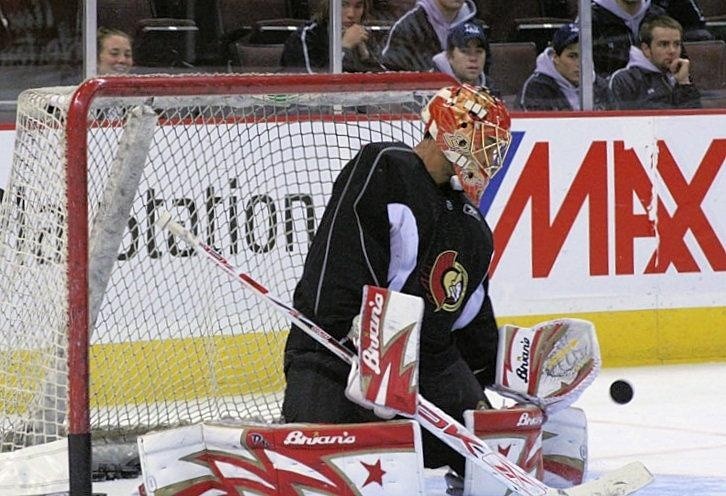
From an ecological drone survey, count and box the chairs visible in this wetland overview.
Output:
[131,1,726,110]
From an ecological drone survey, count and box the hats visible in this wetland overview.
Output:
[448,22,487,50]
[552,23,581,55]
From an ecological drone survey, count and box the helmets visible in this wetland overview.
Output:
[420,82,512,206]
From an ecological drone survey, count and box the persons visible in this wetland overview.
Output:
[514,22,620,112]
[652,0,712,41]
[382,0,493,75]
[402,23,502,114]
[281,83,588,495]
[574,0,689,78]
[60,26,154,119]
[608,15,702,110]
[280,0,387,113]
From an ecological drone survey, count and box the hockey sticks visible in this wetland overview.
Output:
[154,210,654,496]
